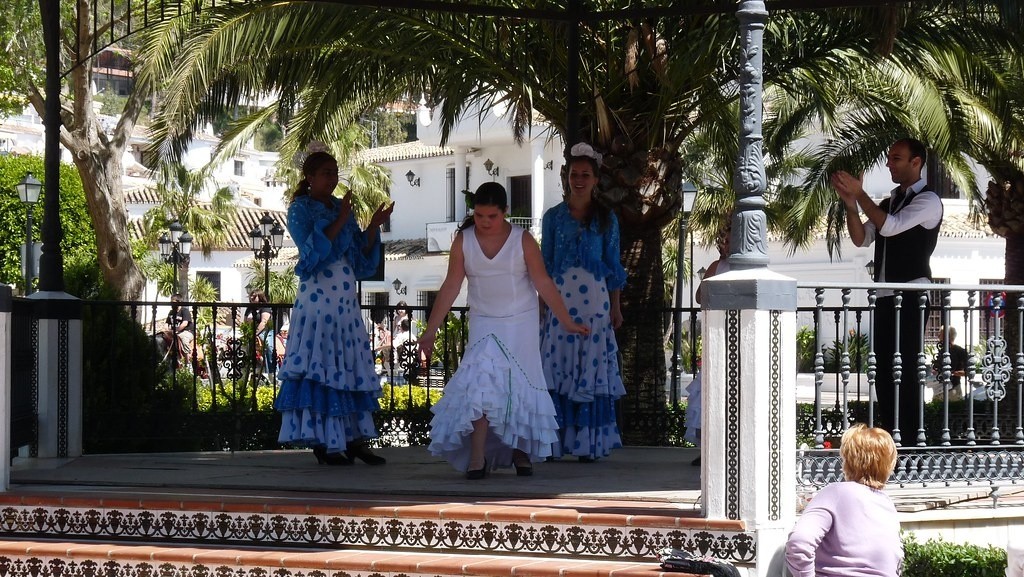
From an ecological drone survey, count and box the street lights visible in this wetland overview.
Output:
[248,210,287,302]
[157,219,194,304]
[15,170,44,297]
[668,179,701,408]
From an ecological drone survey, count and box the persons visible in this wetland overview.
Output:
[244,289,278,388]
[537,154,630,462]
[165,294,195,369]
[415,182,591,480]
[830,137,944,475]
[367,300,427,378]
[274,151,396,467]
[683,225,733,467]
[785,422,906,577]
[932,325,971,402]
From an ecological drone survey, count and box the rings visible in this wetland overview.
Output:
[427,348,431,350]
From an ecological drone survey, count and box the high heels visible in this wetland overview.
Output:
[313,444,353,466]
[466,456,487,480]
[344,442,387,465]
[512,448,534,476]
